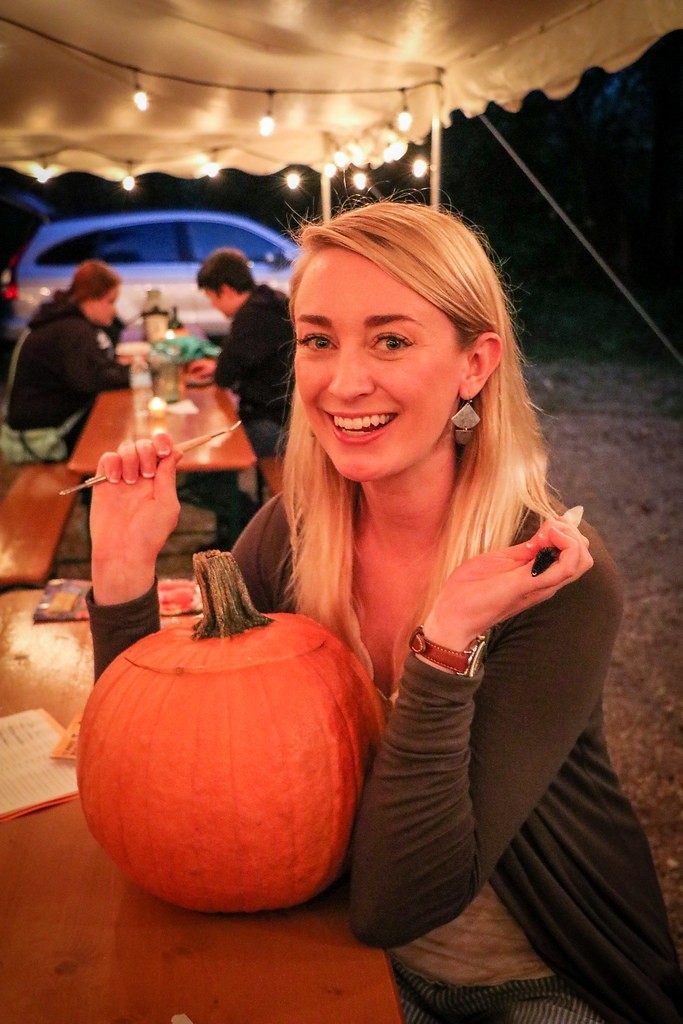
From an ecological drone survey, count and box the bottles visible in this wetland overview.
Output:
[168,306,181,329]
[129,354,154,418]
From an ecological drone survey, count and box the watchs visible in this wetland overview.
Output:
[410,623,489,679]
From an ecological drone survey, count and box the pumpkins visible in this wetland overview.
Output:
[72,546,388,914]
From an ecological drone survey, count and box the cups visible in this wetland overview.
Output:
[159,360,180,402]
[142,307,168,344]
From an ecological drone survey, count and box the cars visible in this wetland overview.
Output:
[0,199,54,271]
[0,209,301,340]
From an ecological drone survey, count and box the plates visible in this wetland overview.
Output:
[114,341,152,356]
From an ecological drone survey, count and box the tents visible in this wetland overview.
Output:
[0,0,683,223]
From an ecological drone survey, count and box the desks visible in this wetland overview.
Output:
[0,588,404,1024]
[68,386,256,547]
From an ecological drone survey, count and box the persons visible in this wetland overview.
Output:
[175,247,297,549]
[0,261,129,503]
[87,190,683,1024]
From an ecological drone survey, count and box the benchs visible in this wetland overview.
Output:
[257,455,284,510]
[0,459,82,588]
